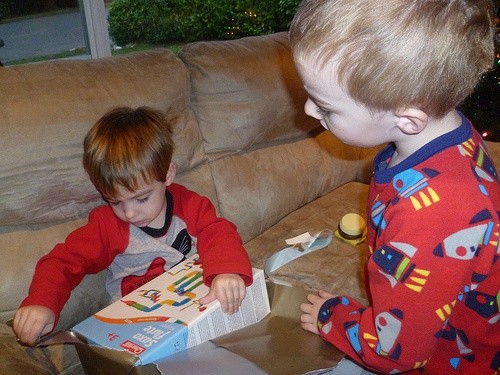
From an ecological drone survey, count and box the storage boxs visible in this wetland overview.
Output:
[74,252,271,375]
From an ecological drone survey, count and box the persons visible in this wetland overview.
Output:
[10,106,254,347]
[289,0,500,375]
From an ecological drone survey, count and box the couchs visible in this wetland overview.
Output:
[0,31,500,375]
